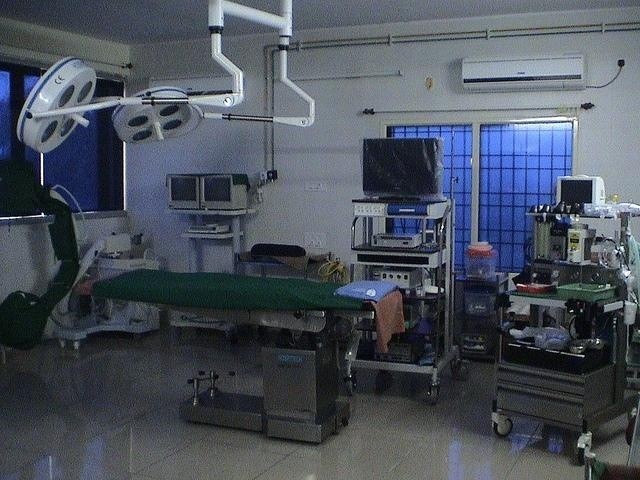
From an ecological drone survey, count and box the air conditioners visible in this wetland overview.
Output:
[461,51,588,92]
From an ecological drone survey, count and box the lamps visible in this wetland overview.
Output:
[14,0,317,155]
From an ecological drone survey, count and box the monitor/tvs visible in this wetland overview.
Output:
[362,137,437,200]
[556,174,605,205]
[166,173,200,210]
[201,174,247,210]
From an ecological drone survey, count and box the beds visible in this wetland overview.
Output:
[90,268,398,334]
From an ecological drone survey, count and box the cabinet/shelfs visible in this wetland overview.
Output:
[168,208,255,273]
[344,200,469,403]
